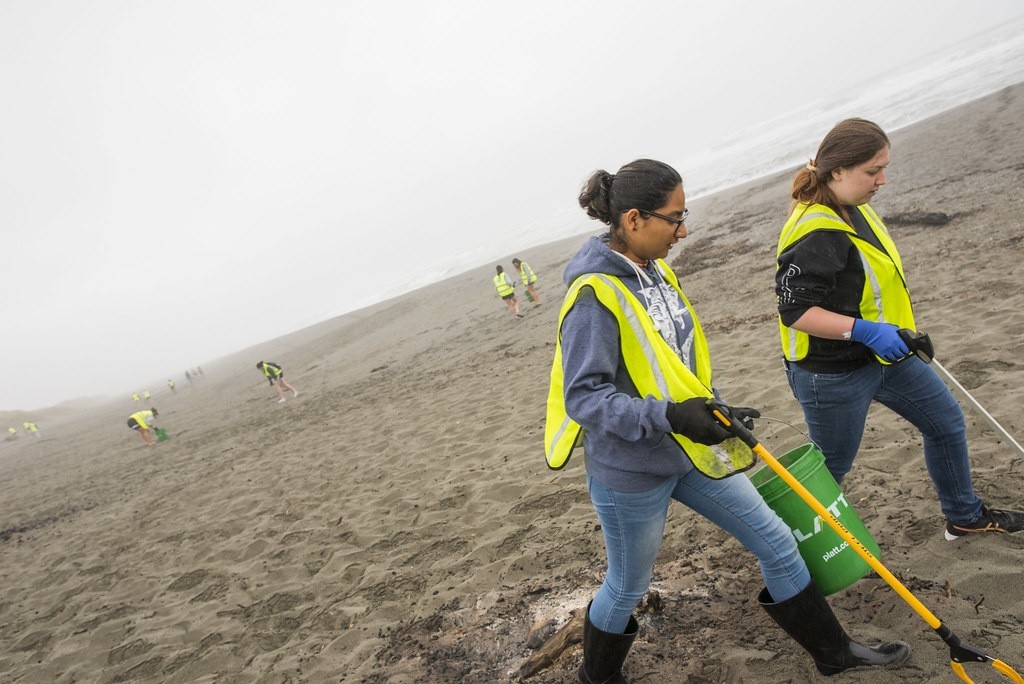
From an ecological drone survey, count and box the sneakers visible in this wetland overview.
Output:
[944,504,1024,541]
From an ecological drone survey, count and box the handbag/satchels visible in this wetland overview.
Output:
[154,428,168,442]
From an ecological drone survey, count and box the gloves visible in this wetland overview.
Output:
[732,405,761,429]
[667,397,737,446]
[850,318,910,363]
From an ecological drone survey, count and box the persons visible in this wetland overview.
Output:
[22,421,38,433]
[132,392,141,403]
[168,379,175,392]
[184,366,203,382]
[256,361,298,403]
[7,427,16,435]
[493,265,524,318]
[543,159,911,684]
[772,117,1024,541]
[512,258,541,305]
[127,407,158,446]
[142,389,151,400]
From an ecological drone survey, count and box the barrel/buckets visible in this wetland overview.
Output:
[746,416,881,595]
[155,428,167,441]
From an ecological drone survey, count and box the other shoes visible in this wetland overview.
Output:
[278,399,286,403]
[294,391,298,397]
[516,314,524,318]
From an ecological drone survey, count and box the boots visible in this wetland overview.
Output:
[757,573,912,676]
[576,599,639,683]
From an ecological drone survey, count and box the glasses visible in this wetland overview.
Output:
[623,208,689,232]
